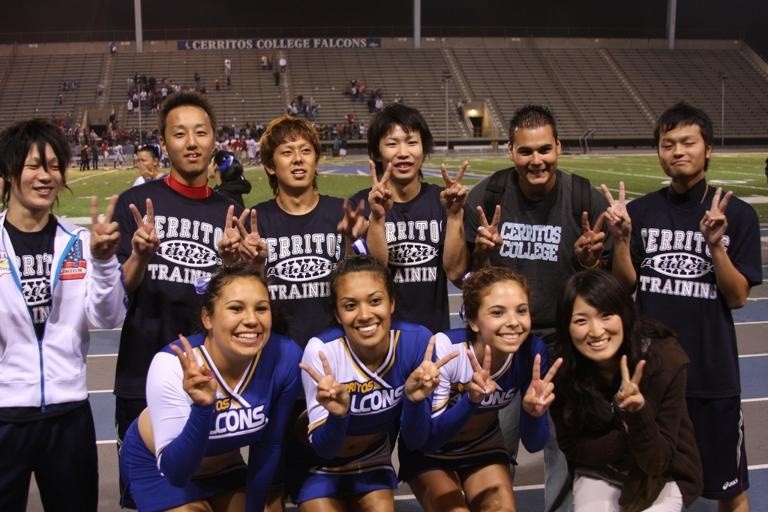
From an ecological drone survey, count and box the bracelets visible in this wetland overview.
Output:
[578,259,599,270]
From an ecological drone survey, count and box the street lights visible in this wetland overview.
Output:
[135,72,144,145]
[442,68,453,148]
[718,70,729,148]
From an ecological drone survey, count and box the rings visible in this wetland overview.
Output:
[476,225,484,231]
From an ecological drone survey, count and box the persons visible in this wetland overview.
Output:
[109,91,250,512]
[287,251,459,512]
[209,150,252,208]
[129,146,167,189]
[110,42,116,53]
[124,57,231,114]
[398,266,564,512]
[601,97,761,511]
[1,116,130,511]
[285,80,401,157]
[453,105,613,512]
[122,264,303,511]
[538,269,705,512]
[215,121,266,164]
[58,80,104,105]
[351,101,469,336]
[261,54,286,86]
[232,115,368,511]
[56,113,168,170]
